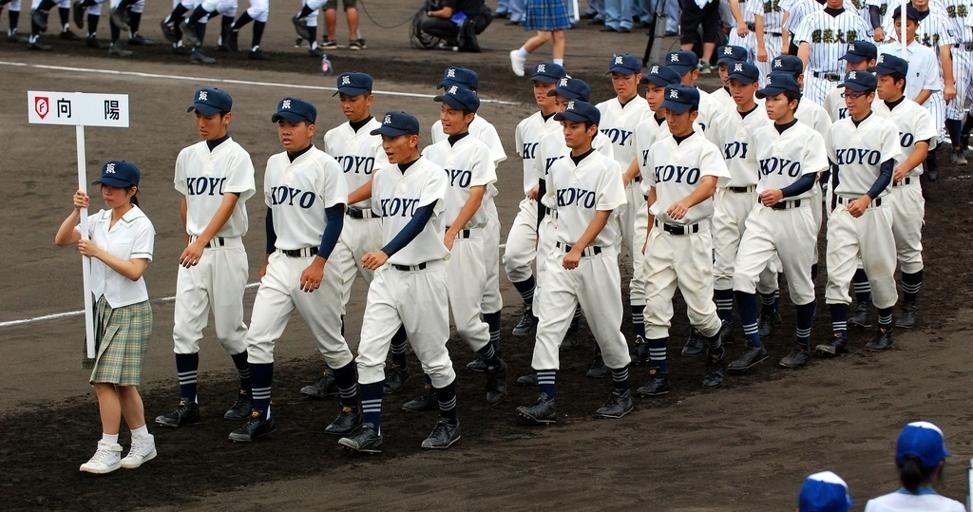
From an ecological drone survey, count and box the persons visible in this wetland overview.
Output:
[635,84,732,397]
[596,54,658,257]
[726,69,830,370]
[300,72,413,397]
[864,422,967,512]
[635,63,711,368]
[155,89,257,428]
[517,77,613,385]
[431,66,507,373]
[502,60,567,338]
[337,111,462,453]
[400,85,509,414]
[795,470,853,512]
[707,63,783,339]
[420,1,663,76]
[766,54,832,284]
[55,162,158,475]
[847,54,939,329]
[826,41,879,123]
[709,46,747,104]
[664,49,726,131]
[815,70,903,357]
[516,99,634,424]
[228,97,363,447]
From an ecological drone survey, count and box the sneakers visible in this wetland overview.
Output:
[59,27,81,41]
[73,1,87,29]
[698,60,711,75]
[777,342,809,369]
[85,34,100,50]
[7,29,28,44]
[518,360,571,387]
[31,7,49,32]
[383,363,411,394]
[24,34,56,50]
[618,27,631,34]
[631,339,650,367]
[179,17,202,47]
[120,434,157,468]
[774,312,785,324]
[128,37,154,45]
[300,368,343,400]
[466,348,504,372]
[189,46,217,64]
[587,18,605,25]
[491,13,507,19]
[160,13,182,42]
[80,440,123,474]
[108,41,134,57]
[155,398,201,427]
[349,39,360,50]
[600,25,617,33]
[110,12,130,32]
[586,344,610,379]
[847,303,872,329]
[928,166,939,181]
[636,367,672,396]
[727,343,770,375]
[317,40,338,51]
[633,21,650,29]
[680,328,707,357]
[512,394,557,427]
[249,46,269,60]
[816,334,849,358]
[757,314,774,338]
[592,385,634,419]
[703,348,725,390]
[309,47,332,60]
[895,302,917,329]
[485,360,509,407]
[358,39,367,50]
[325,404,363,435]
[401,394,439,413]
[292,12,311,40]
[863,325,894,352]
[451,44,459,52]
[512,303,537,337]
[950,149,966,167]
[422,415,461,449]
[438,41,450,48]
[225,389,254,421]
[505,21,518,26]
[720,321,735,345]
[664,31,678,37]
[338,424,384,456]
[580,14,593,21]
[172,41,192,56]
[510,50,527,76]
[228,407,278,443]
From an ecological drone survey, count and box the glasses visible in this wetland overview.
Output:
[841,92,866,98]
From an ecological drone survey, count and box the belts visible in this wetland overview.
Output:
[444,228,469,239]
[655,219,698,236]
[189,236,224,248]
[813,72,840,82]
[727,186,757,193]
[556,242,601,257]
[387,263,426,272]
[764,31,782,36]
[277,246,317,257]
[893,175,910,188]
[838,197,881,209]
[746,22,756,31]
[345,207,381,219]
[758,195,801,210]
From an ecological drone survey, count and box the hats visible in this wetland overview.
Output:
[437,67,479,91]
[755,70,801,99]
[837,70,877,91]
[370,111,419,136]
[604,53,642,76]
[553,99,600,125]
[91,159,140,187]
[717,46,747,67]
[896,421,952,467]
[838,40,877,64]
[187,87,232,115]
[799,471,853,512]
[332,71,373,97]
[640,63,681,87]
[547,76,592,102]
[892,5,919,22]
[873,53,908,76]
[658,84,699,113]
[766,55,803,80]
[664,50,698,75]
[725,61,759,84]
[530,63,565,83]
[434,83,480,114]
[271,97,316,123]
